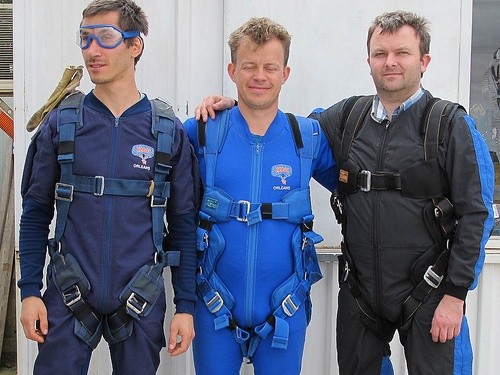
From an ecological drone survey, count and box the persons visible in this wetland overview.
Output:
[194,10,494,375]
[18,1,199,374]
[183,17,338,375]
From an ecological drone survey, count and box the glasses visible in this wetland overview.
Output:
[74,24,138,50]
